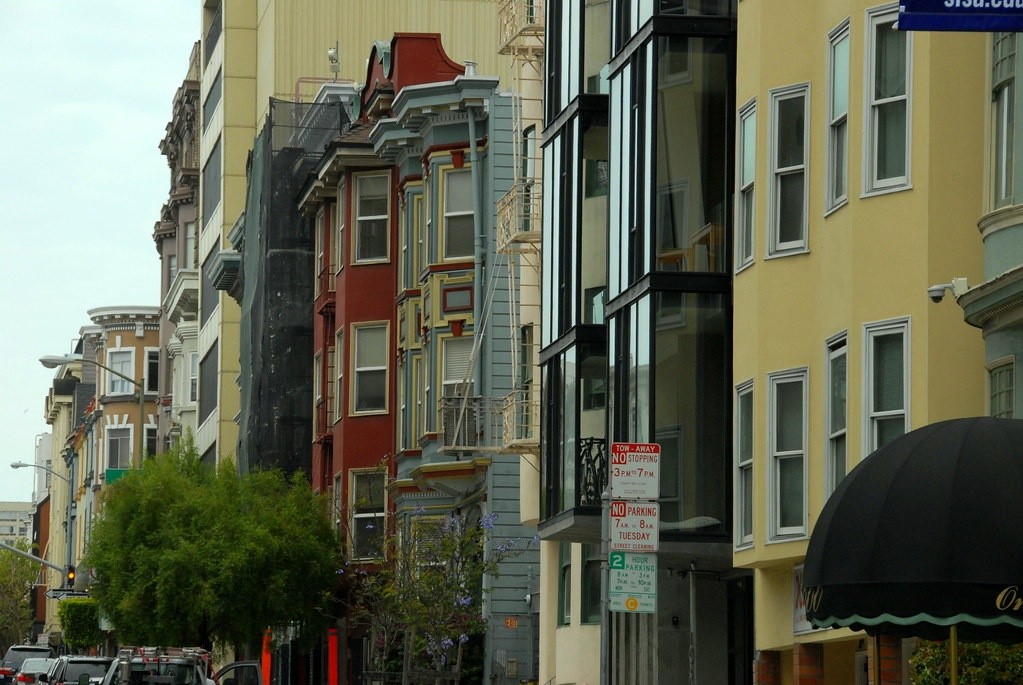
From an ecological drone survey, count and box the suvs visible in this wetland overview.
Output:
[38,653,122,685]
[0,643,56,685]
[77,647,262,685]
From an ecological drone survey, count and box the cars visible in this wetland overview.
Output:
[10,657,57,685]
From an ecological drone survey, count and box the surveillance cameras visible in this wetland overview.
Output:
[927,286,946,303]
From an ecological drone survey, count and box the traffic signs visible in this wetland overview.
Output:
[44,588,75,599]
[56,591,88,601]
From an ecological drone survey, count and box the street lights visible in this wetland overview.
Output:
[9,462,74,588]
[38,354,145,470]
